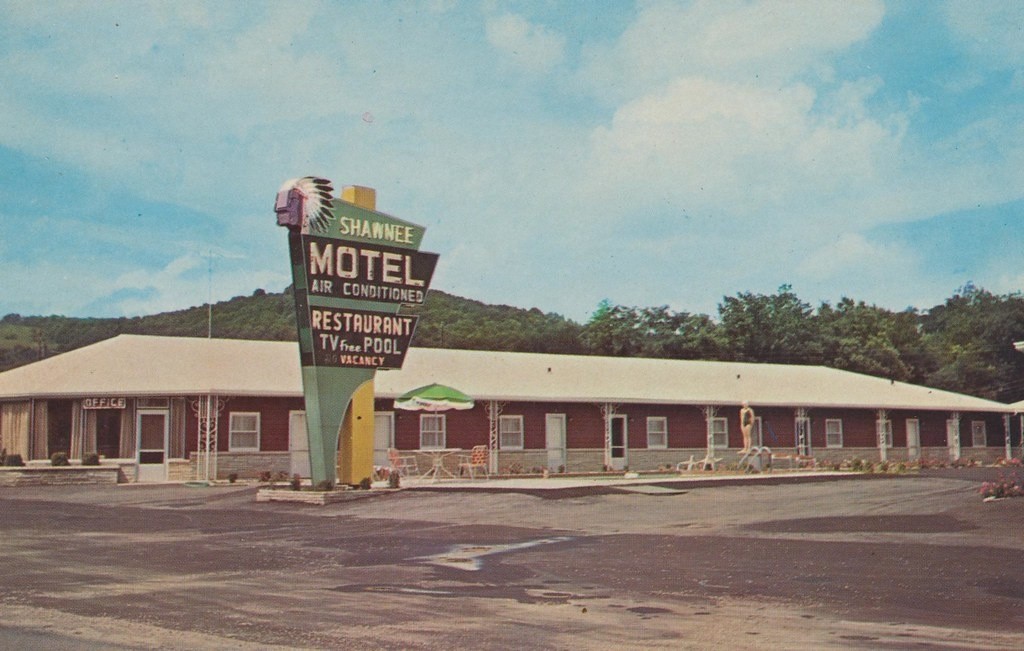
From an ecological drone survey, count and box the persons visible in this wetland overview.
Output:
[735,400,755,454]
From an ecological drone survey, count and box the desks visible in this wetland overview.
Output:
[414,447,463,484]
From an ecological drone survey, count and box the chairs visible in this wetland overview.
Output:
[457,443,489,480]
[386,447,419,478]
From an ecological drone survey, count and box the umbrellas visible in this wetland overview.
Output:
[392,382,475,450]
